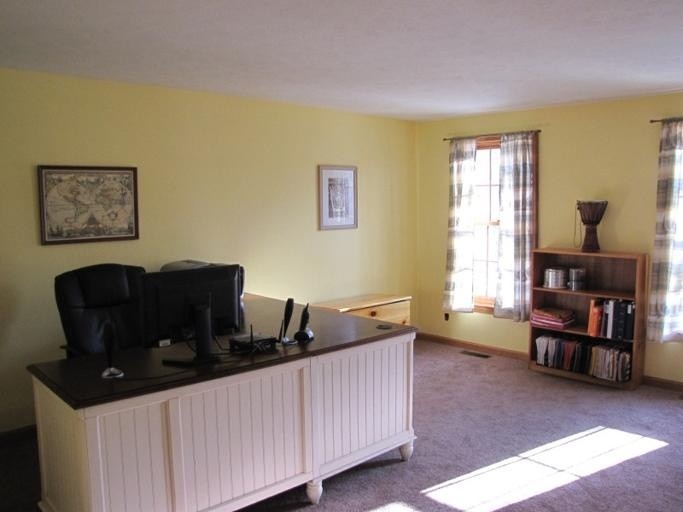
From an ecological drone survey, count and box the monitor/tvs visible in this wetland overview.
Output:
[139,264,242,366]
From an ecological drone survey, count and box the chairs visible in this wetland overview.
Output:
[54,263,147,355]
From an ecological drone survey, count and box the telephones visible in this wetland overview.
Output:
[293,303,314,343]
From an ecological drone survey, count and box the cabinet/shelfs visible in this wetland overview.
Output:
[528,246,651,392]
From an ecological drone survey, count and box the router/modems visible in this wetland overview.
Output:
[228,319,284,355]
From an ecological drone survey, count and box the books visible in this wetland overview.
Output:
[534,334,630,383]
[530,307,576,330]
[585,296,634,340]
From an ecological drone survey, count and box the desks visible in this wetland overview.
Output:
[25,295,417,512]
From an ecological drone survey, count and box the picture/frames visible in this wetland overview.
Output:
[316,166,358,232]
[38,165,139,245]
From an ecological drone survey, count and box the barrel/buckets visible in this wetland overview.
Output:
[543,267,567,289]
